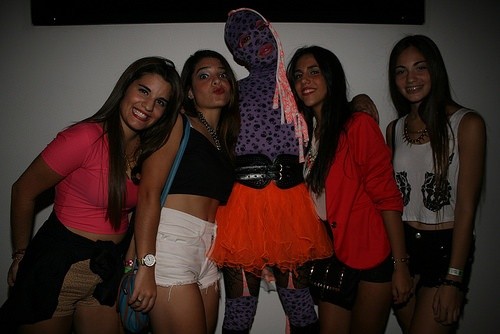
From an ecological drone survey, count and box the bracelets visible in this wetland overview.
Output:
[11,247,28,259]
[391,254,411,267]
[442,278,467,289]
[448,266,466,277]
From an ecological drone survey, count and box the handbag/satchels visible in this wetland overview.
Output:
[118,264,151,334]
[307,257,362,309]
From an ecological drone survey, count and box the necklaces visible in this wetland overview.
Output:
[402,110,431,146]
[195,108,224,152]
[125,148,141,170]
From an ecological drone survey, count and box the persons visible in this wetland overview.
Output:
[286,45,413,334]
[205,7,380,334]
[386,33,488,334]
[0,56,185,334]
[128,50,238,334]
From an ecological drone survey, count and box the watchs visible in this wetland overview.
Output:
[139,252,157,266]
